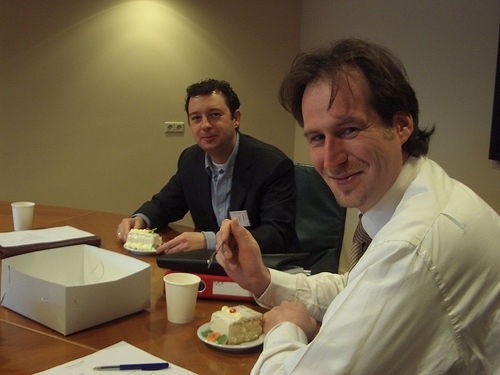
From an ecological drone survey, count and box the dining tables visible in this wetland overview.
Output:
[0,199,321,374]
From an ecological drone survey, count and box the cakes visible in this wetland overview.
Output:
[201,304,264,345]
[124,228,166,250]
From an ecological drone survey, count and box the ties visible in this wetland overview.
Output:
[349,222,372,274]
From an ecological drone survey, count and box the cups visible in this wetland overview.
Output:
[163,273,201,324]
[11,201,36,231]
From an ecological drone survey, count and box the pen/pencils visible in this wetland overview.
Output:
[93,362,170,371]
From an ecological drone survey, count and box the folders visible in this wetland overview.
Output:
[163,270,256,304]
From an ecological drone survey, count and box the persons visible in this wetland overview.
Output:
[214,37,499,375]
[118,79,308,269]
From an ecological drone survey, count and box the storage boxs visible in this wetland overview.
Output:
[0,244,151,337]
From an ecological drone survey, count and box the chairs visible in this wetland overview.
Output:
[294,164,347,278]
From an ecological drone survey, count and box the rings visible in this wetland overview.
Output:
[118,232,120,236]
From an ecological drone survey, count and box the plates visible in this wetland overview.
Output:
[196,322,265,351]
[123,242,165,256]
